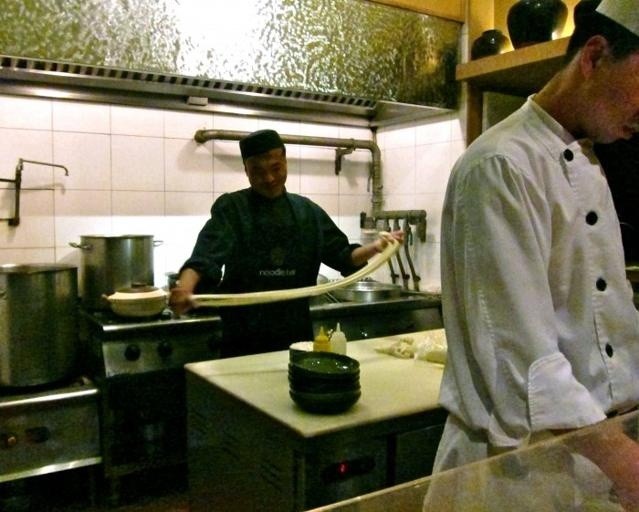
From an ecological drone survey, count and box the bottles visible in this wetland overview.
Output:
[314,323,346,357]
[468,0,567,62]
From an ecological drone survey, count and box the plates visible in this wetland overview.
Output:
[287,340,361,413]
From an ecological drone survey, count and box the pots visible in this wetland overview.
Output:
[0,235,164,391]
[332,280,403,302]
[102,283,172,317]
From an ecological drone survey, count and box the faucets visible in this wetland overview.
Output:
[1,158,69,224]
[22,182,67,195]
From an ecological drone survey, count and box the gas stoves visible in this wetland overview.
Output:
[75,307,224,384]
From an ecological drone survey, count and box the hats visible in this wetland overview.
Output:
[239,130,285,160]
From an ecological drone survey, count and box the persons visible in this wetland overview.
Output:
[170,130,404,357]
[422,0,639,512]
